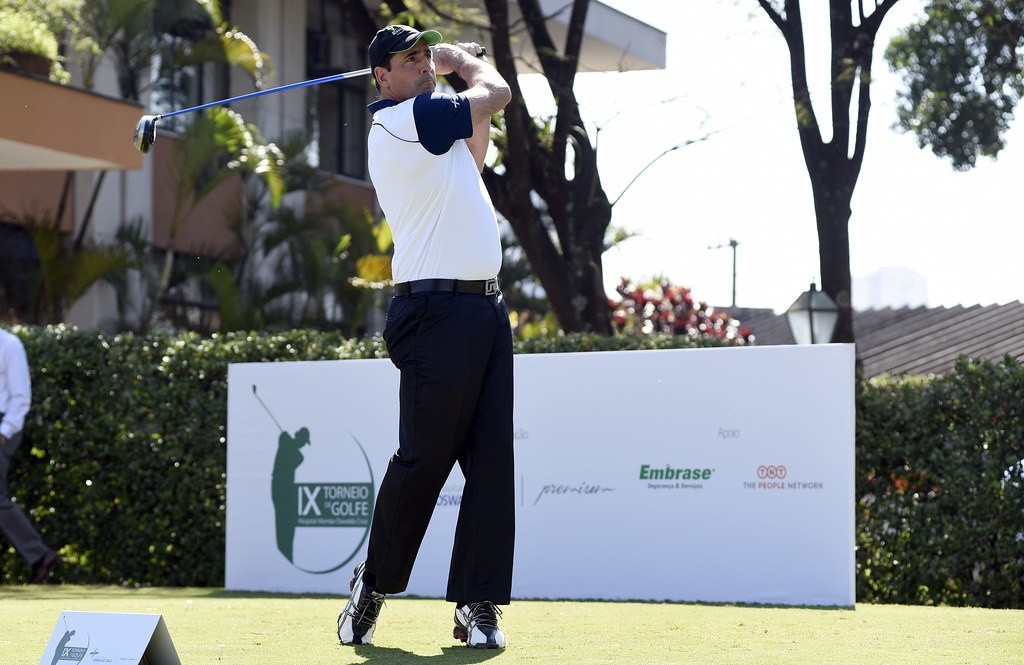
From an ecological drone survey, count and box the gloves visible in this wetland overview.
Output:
[454,38,485,61]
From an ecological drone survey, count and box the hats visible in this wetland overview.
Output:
[368,24,442,70]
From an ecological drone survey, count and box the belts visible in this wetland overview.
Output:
[394,278,498,296]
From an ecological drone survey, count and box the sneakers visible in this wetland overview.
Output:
[453,601,506,649]
[337,561,385,645]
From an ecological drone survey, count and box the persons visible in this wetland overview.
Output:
[337,24,516,648]
[0,326,66,584]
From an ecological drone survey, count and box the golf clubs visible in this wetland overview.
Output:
[136,45,487,153]
[252,384,283,432]
